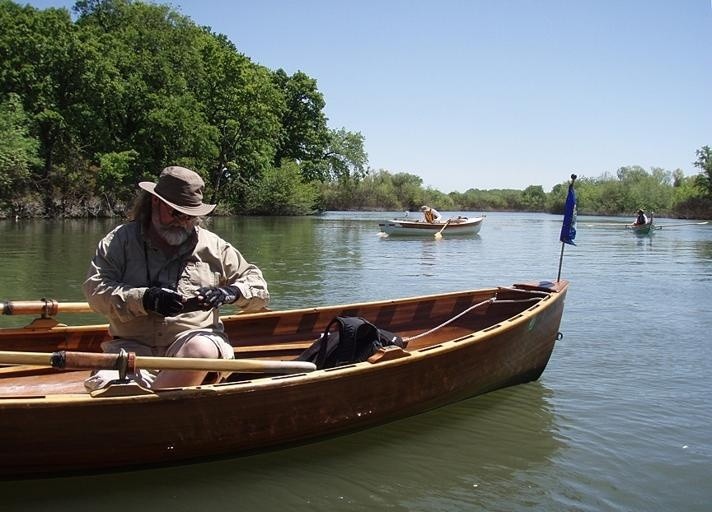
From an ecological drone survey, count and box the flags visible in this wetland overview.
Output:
[560,184,578,247]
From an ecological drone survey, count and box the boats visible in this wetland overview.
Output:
[376,213,488,238]
[0,276,575,487]
[633,212,655,234]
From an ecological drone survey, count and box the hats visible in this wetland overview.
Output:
[139,166,217,217]
[419,205,429,212]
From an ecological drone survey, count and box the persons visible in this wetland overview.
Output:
[419,205,441,223]
[82,166,271,393]
[636,209,648,225]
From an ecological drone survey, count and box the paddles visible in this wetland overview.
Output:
[586,224,636,228]
[433,220,449,237]
[653,222,708,225]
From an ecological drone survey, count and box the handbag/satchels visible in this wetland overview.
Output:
[298,316,381,367]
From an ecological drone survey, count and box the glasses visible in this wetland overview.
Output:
[162,204,198,220]
